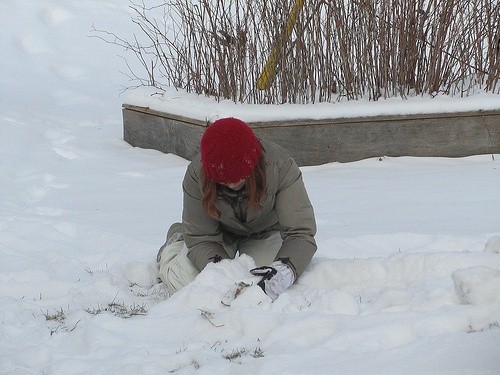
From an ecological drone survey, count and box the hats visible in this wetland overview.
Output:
[200,117,260,183]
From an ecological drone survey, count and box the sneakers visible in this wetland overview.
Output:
[157,223,184,263]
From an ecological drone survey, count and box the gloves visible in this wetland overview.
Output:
[250,260,296,302]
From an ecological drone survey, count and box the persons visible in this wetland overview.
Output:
[155,117,318,303]
